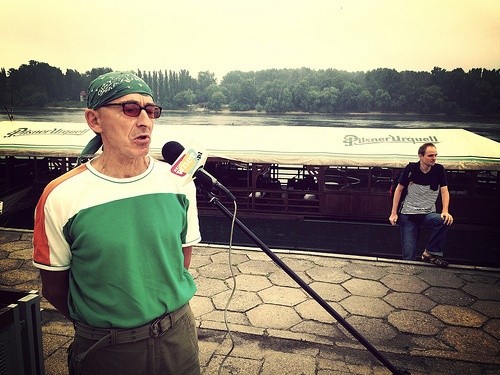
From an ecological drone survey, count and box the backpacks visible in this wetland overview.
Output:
[389,162,415,215]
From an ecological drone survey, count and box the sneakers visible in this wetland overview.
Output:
[422,251,449,267]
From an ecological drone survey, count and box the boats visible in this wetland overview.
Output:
[0,120,500,268]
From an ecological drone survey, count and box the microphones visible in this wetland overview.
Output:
[162,141,236,201]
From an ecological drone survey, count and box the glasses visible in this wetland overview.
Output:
[100,102,162,119]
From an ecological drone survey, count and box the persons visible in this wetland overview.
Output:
[389,143,454,268]
[32,70,202,375]
